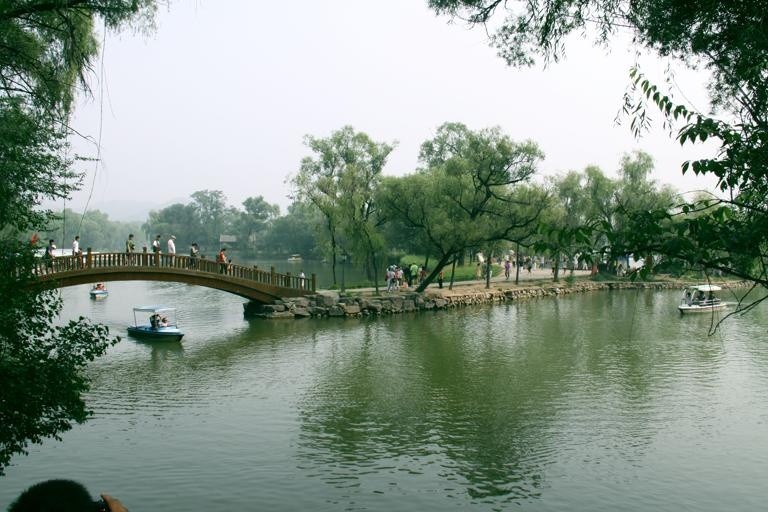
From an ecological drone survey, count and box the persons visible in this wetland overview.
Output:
[167,235,177,268]
[153,235,160,266]
[95,284,106,290]
[218,248,233,276]
[151,314,168,329]
[125,233,135,265]
[686,291,717,305]
[7,478,131,512]
[385,262,428,293]
[298,269,305,289]
[185,243,199,271]
[438,271,443,288]
[29,232,82,275]
[475,249,629,281]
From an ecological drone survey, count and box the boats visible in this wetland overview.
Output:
[126,303,185,344]
[287,253,304,262]
[676,283,728,314]
[89,287,109,302]
